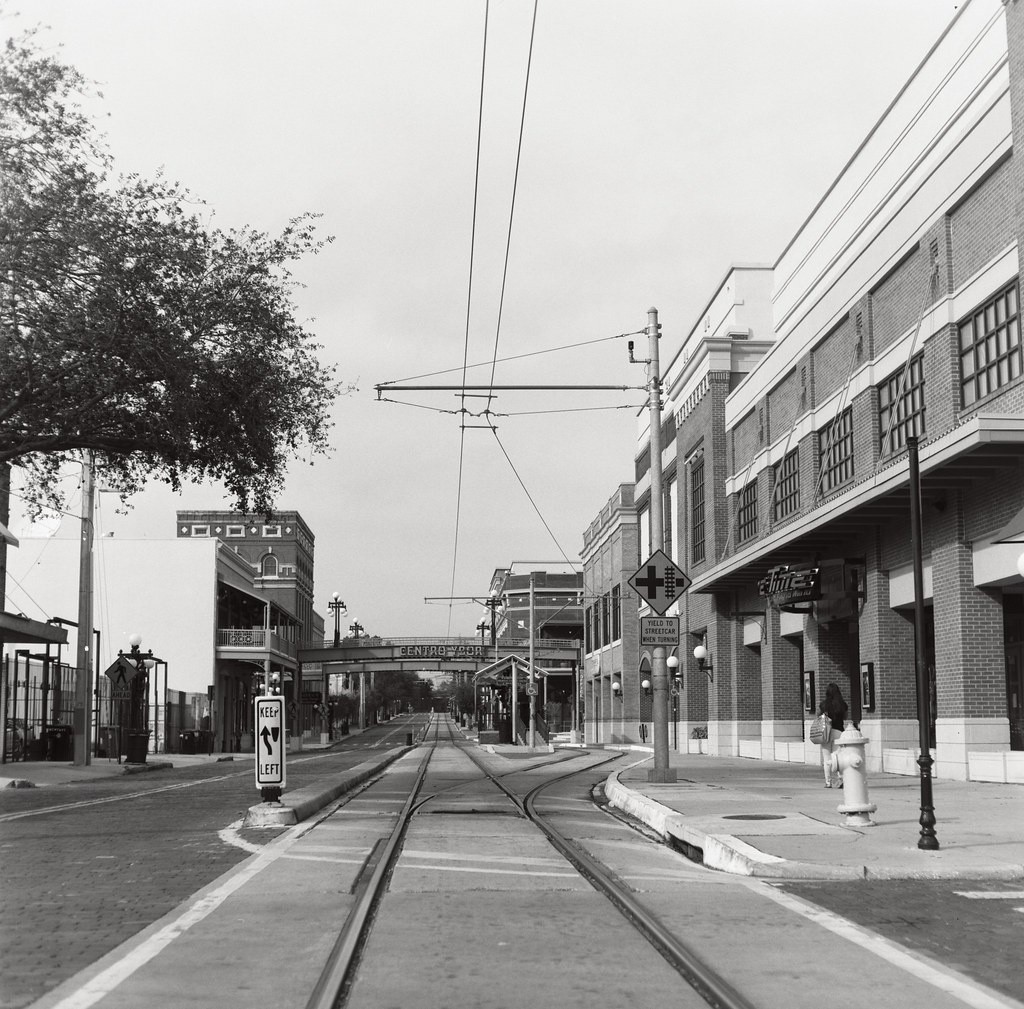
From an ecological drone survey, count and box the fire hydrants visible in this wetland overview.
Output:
[824,725,878,829]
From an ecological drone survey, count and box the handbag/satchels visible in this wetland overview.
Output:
[810,701,832,744]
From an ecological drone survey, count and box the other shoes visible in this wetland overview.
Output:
[837,781,843,788]
[824,784,832,788]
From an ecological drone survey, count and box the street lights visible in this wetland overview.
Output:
[327,592,348,649]
[474,617,492,646]
[327,700,338,741]
[349,617,364,638]
[118,633,153,730]
[483,590,504,647]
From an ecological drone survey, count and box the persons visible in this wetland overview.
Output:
[818,682,848,789]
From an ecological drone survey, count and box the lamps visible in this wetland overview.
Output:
[611,682,623,703]
[667,655,683,690]
[693,646,714,684]
[641,679,654,702]
